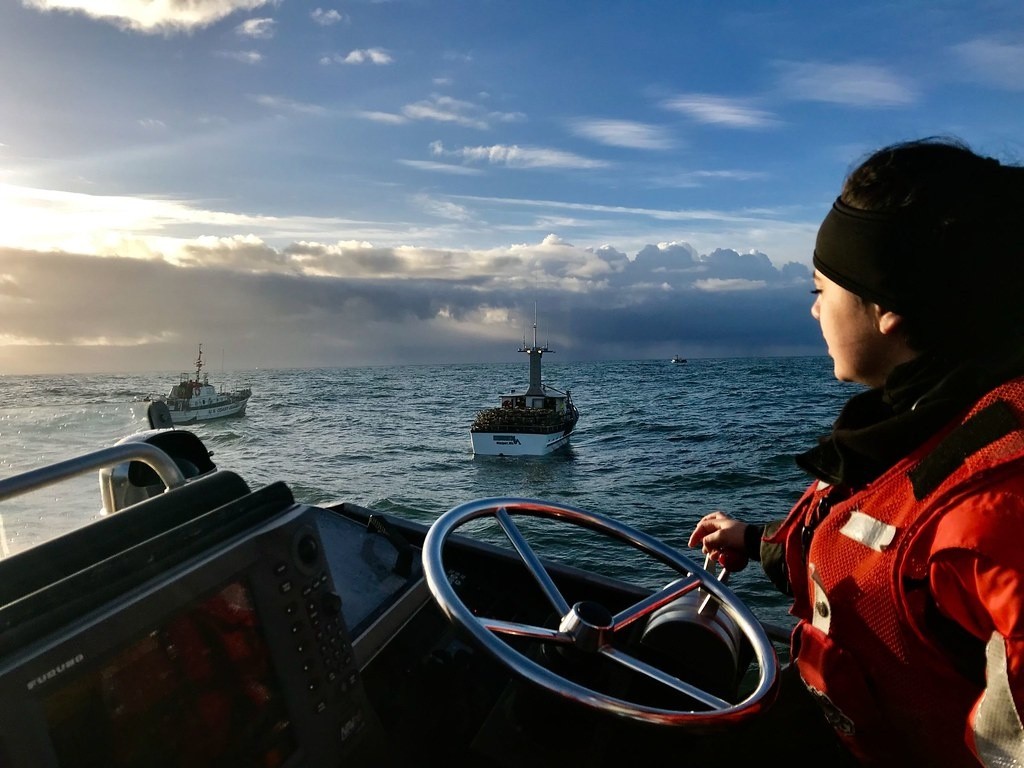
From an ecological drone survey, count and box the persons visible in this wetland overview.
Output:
[689,143,1024,768]
[471,407,564,435]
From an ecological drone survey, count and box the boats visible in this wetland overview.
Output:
[468,301,579,458]
[671,355,687,364]
[159,342,253,426]
